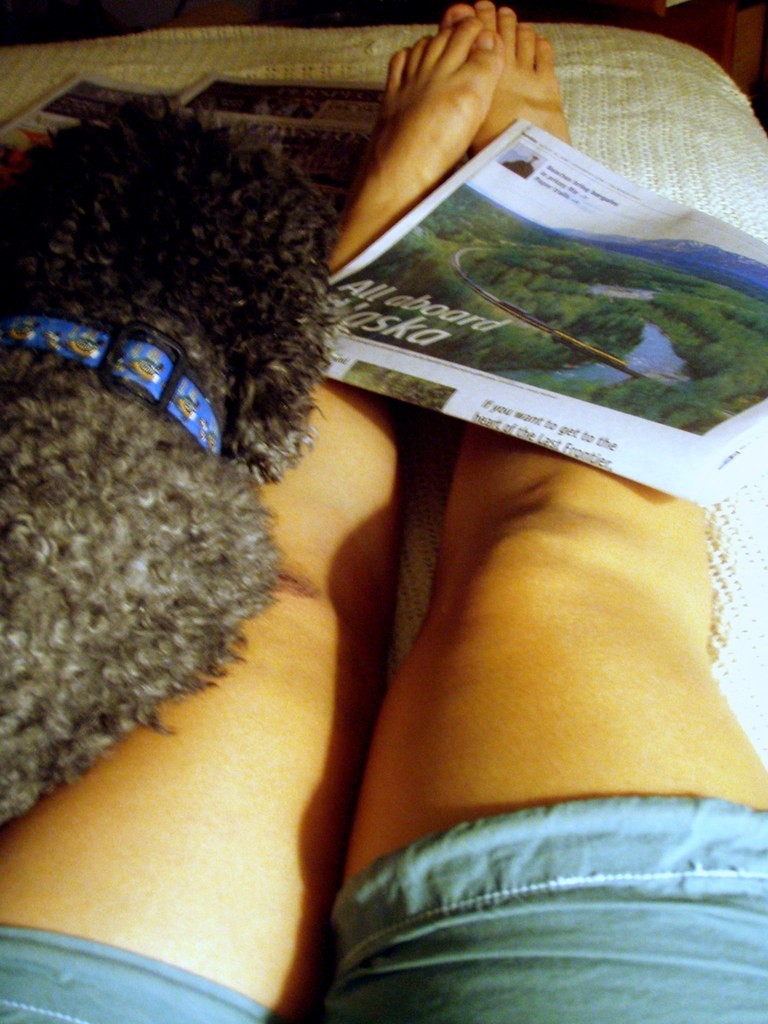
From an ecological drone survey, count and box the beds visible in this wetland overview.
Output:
[0,17,768,781]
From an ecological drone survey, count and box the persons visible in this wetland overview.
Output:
[0,1,766,1024]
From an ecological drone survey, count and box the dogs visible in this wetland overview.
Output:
[0,91,364,831]
